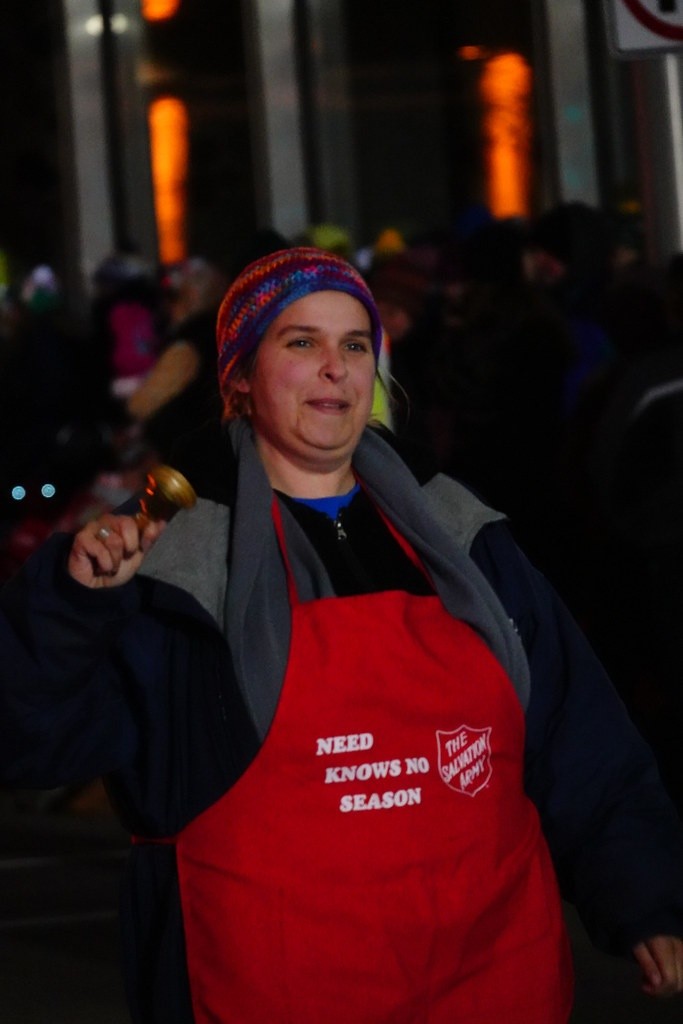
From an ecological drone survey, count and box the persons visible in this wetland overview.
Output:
[0,249,683,1024]
[0,202,683,813]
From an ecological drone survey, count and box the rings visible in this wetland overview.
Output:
[97,527,112,539]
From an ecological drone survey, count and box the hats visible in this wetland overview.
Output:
[216,246,408,401]
